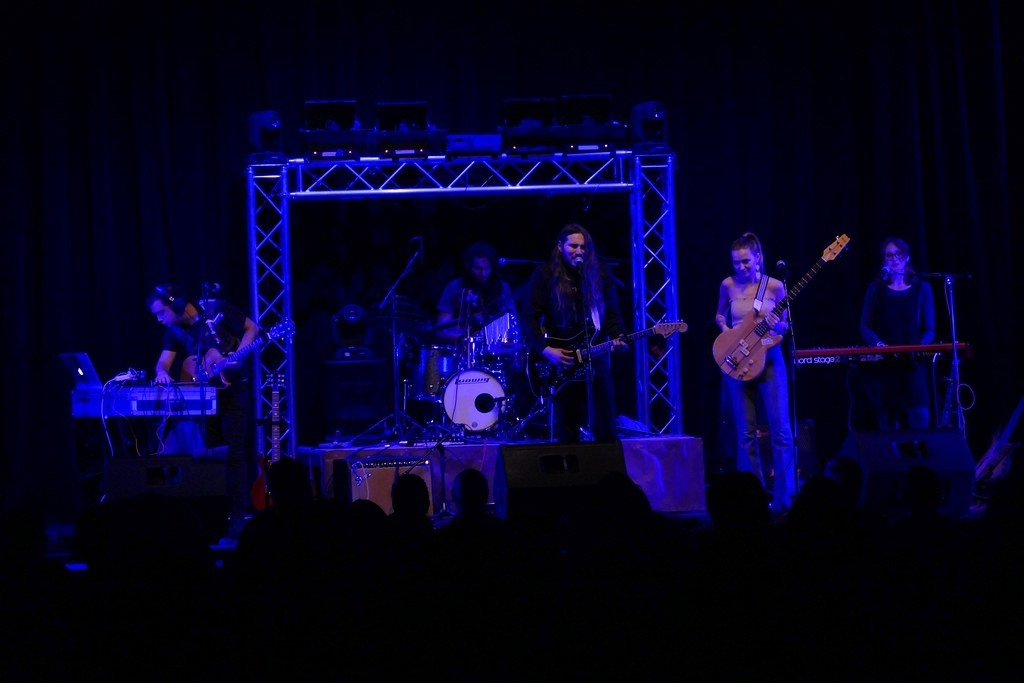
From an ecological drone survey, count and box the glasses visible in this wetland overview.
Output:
[885,251,904,260]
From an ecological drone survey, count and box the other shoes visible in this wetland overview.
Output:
[134,448,157,458]
[769,496,793,510]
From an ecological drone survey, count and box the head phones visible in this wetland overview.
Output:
[154,286,186,314]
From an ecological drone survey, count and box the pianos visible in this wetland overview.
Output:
[789,339,966,496]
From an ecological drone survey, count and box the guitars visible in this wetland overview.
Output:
[712,234,850,382]
[975,395,1024,484]
[251,370,293,511]
[180,315,297,390]
[525,319,688,401]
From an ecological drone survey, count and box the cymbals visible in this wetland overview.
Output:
[375,293,417,319]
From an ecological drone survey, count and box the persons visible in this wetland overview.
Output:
[0,457,1024,683]
[147,284,260,525]
[523,220,630,442]
[710,232,800,517]
[436,242,520,346]
[861,236,937,432]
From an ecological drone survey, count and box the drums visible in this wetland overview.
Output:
[400,343,467,402]
[439,368,507,434]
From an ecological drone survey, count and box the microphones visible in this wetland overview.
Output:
[881,266,890,281]
[498,258,528,266]
[777,260,790,276]
[418,236,425,264]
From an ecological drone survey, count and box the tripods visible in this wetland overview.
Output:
[348,250,435,446]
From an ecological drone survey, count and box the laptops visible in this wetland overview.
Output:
[60,352,103,387]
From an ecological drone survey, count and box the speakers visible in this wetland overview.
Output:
[493,439,627,521]
[833,430,978,513]
[108,457,234,543]
[331,456,436,521]
